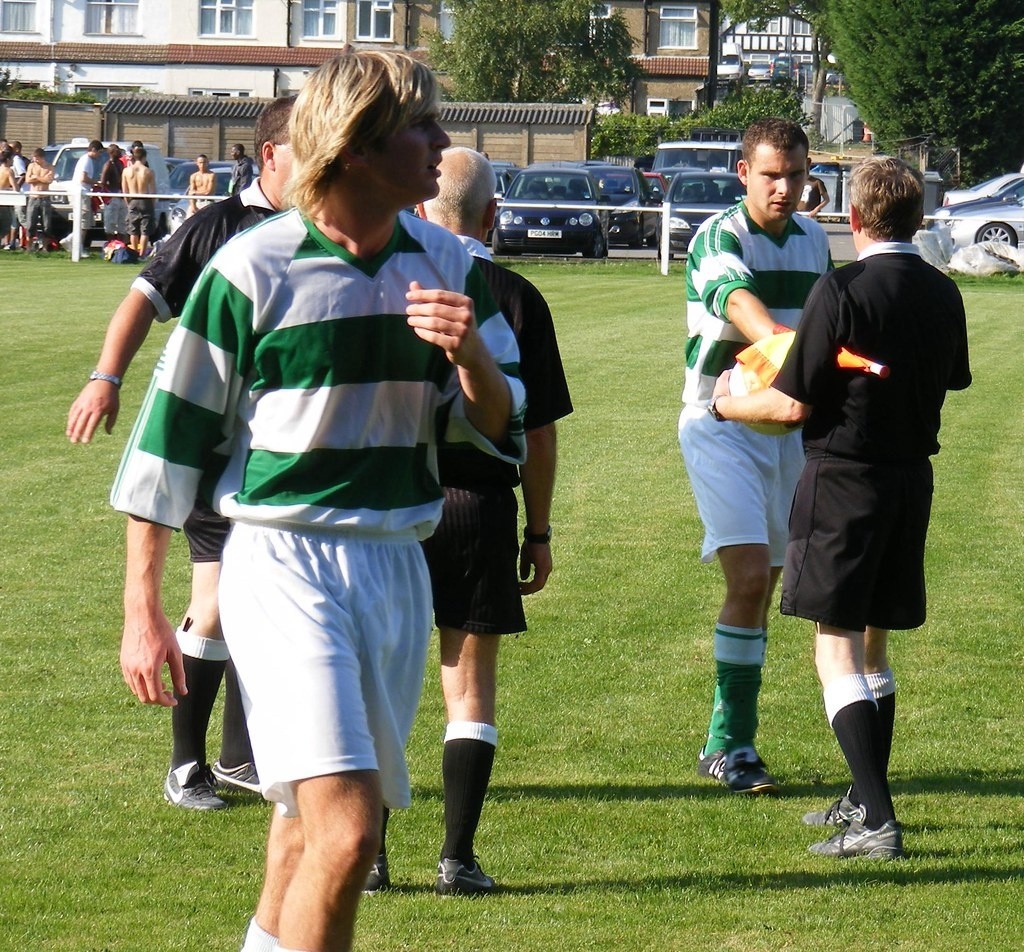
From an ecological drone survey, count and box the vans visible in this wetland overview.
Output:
[651,139,744,195]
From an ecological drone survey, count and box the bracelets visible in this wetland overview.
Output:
[89,370,122,387]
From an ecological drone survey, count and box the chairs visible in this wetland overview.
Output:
[552,186,567,195]
[706,183,721,196]
[527,180,547,193]
[603,180,618,189]
[682,185,702,199]
[723,185,739,197]
[709,151,726,166]
[568,179,589,193]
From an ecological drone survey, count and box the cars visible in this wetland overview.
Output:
[926,172,1024,253]
[20,137,261,250]
[483,159,748,260]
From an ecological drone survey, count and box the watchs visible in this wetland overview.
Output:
[706,393,731,423]
[524,524,553,544]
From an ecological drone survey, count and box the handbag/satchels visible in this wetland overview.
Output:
[101,239,139,263]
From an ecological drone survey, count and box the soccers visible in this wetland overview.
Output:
[728,360,808,438]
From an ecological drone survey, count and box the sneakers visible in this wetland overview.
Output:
[437,854,494,897]
[802,784,859,825]
[163,761,225,810]
[698,744,778,794]
[211,758,262,793]
[362,854,390,896]
[808,804,902,859]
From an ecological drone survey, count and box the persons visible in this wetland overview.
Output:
[112,51,515,952]
[63,93,305,814]
[100,144,128,249]
[23,147,56,253]
[673,111,836,800]
[227,144,256,197]
[0,138,33,252]
[362,144,575,899]
[185,154,217,221]
[121,148,156,259]
[796,153,832,223]
[124,140,150,169]
[59,140,105,258]
[706,151,974,855]
